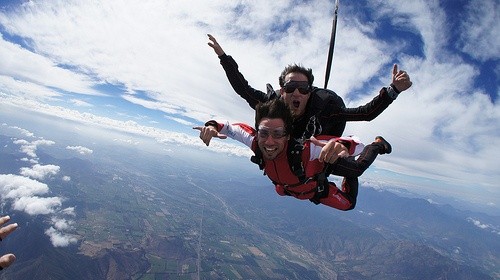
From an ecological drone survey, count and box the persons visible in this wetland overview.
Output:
[191,99,365,211]
[207,34,413,177]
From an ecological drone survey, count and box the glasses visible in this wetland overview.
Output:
[284,81,310,94]
[256,129,288,139]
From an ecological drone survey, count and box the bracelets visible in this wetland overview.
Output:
[387,86,398,100]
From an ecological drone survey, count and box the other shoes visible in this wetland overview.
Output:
[376,136,392,154]
